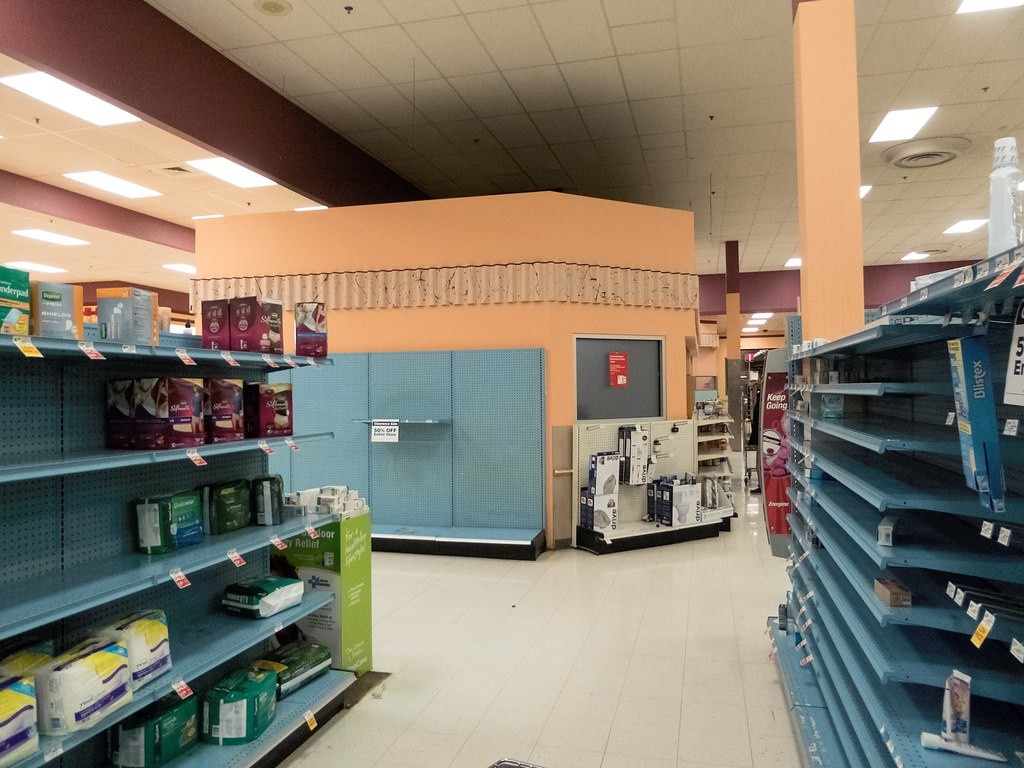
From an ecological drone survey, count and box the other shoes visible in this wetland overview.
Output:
[750,488,761,494]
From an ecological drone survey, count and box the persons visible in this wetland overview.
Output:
[748,390,761,495]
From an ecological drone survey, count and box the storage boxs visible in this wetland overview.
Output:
[285,485,370,522]
[873,576,912,607]
[778,590,805,648]
[876,515,901,547]
[579,424,702,540]
[947,334,1007,513]
[103,376,293,450]
[270,511,373,678]
[792,337,867,549]
[0,265,328,358]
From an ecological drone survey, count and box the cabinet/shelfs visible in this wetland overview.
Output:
[571,419,723,556]
[691,405,738,532]
[0,322,358,768]
[767,243,1024,768]
